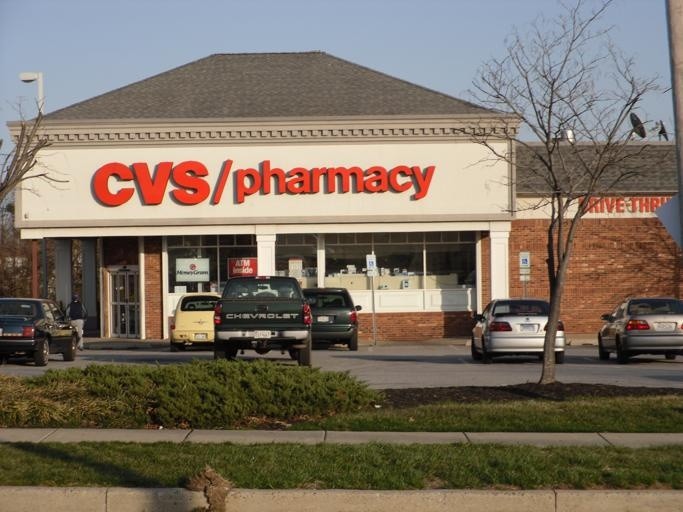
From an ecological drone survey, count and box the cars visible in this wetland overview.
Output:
[471,298,683,363]
[1,298,80,366]
[169,275,362,366]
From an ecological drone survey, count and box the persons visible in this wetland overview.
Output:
[65,294,88,352]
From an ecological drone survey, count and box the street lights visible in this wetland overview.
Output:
[20,70,48,297]
